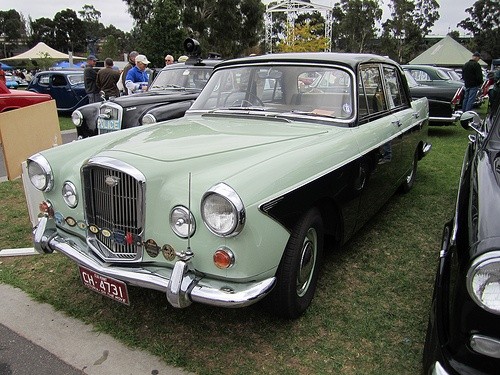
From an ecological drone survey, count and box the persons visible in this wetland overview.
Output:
[0,64,36,86]
[462,52,484,113]
[84,51,189,104]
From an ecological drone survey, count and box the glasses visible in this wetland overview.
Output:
[165,59,170,61]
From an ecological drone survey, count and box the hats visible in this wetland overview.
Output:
[178,55,189,62]
[135,55,151,64]
[86,55,99,61]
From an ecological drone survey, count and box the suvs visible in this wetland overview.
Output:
[0,75,53,113]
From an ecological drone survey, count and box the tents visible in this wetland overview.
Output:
[0,42,87,69]
[407,35,488,67]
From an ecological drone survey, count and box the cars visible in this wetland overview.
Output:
[423,80,500,375]
[72,37,281,141]
[25,71,88,116]
[309,56,492,126]
[5,75,29,90]
[19,53,430,321]
